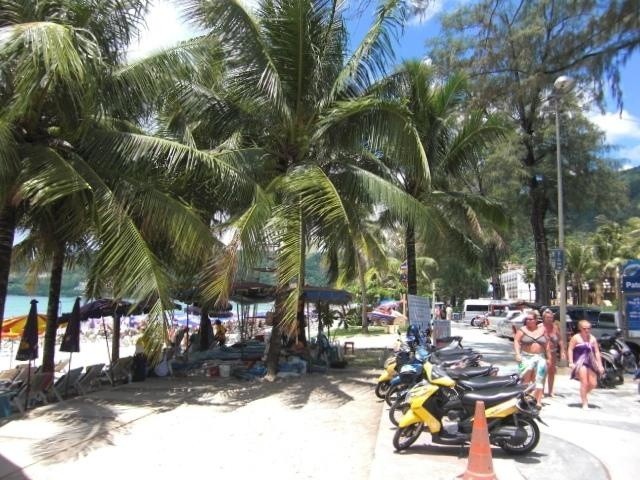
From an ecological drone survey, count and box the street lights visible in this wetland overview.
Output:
[555,76,574,366]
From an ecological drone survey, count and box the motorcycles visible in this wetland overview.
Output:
[597,328,637,388]
[375,324,550,455]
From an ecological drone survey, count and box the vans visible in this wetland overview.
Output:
[463,299,510,326]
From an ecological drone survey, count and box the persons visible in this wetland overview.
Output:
[433,302,454,322]
[536,308,566,398]
[213,320,227,347]
[513,310,553,408]
[167,325,191,360]
[566,319,605,409]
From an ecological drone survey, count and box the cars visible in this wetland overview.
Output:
[474,302,625,341]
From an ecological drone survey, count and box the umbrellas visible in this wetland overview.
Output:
[182,306,234,320]
[227,278,354,362]
[1,310,71,369]
[64,299,134,389]
[15,299,39,415]
[193,296,233,312]
[58,298,81,400]
[121,291,182,319]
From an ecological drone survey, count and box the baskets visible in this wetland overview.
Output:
[399,364,423,383]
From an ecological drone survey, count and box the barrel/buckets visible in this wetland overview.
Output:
[218,364,231,377]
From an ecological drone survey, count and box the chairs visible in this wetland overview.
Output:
[0,354,133,415]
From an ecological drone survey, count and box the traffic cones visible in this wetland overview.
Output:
[452,400,499,480]
